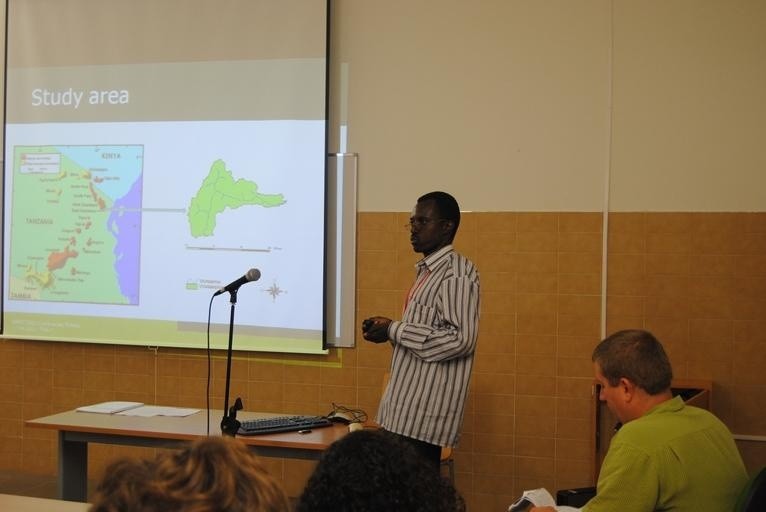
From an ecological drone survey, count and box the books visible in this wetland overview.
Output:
[508,488,579,512]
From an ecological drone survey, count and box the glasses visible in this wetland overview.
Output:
[403,219,448,231]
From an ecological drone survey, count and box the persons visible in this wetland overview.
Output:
[528,330,748,512]
[94,433,291,510]
[363,192,480,476]
[294,429,466,511]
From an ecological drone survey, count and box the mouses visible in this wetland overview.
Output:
[328,412,352,423]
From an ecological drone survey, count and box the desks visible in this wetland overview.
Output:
[23,398,376,506]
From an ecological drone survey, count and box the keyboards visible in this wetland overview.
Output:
[236,415,333,436]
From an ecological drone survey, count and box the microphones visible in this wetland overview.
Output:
[214,269,261,296]
